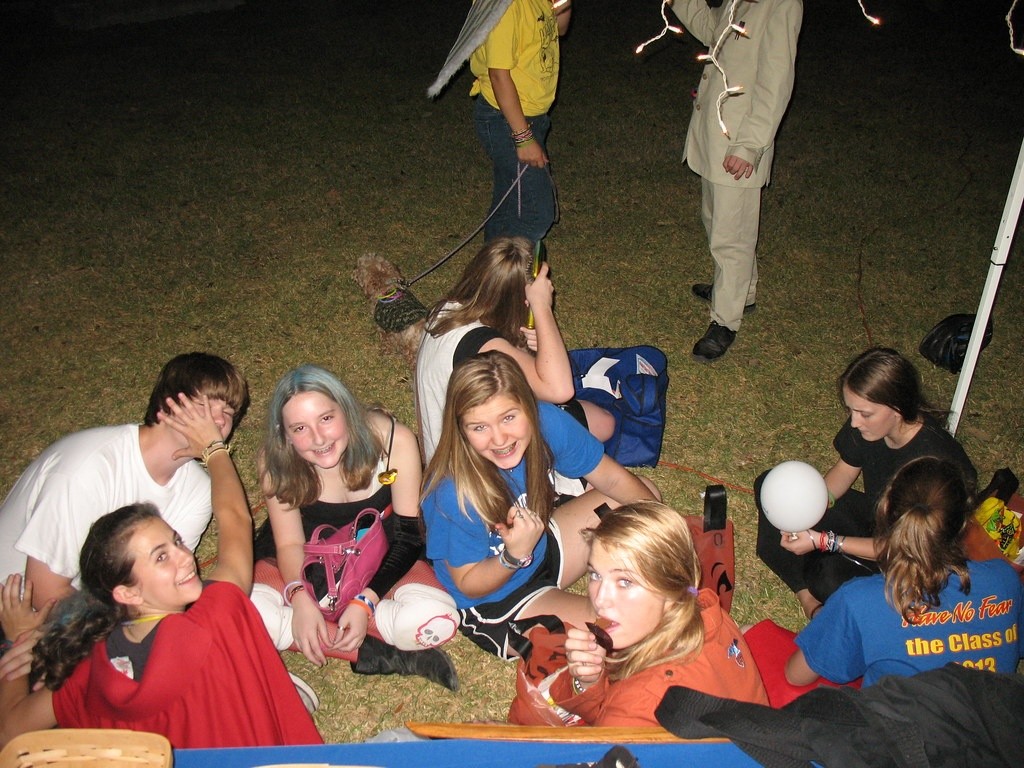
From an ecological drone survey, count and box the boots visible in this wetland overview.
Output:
[350,633,457,691]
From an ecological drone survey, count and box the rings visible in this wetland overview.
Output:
[512,510,524,519]
[337,626,346,631]
[530,511,537,517]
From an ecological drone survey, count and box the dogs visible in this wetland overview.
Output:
[349,253,428,370]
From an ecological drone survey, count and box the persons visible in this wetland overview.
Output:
[0,351,248,623]
[419,350,662,662]
[550,500,770,727]
[0,391,325,752]
[250,365,461,694]
[784,453,1024,692]
[415,235,616,507]
[665,0,803,363]
[755,347,978,624]
[427,0,571,244]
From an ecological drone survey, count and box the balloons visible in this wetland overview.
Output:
[760,461,828,533]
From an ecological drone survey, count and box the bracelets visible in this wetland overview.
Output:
[283,581,304,606]
[807,531,846,553]
[201,440,231,471]
[572,677,586,695]
[499,549,520,570]
[350,594,375,615]
[511,123,535,147]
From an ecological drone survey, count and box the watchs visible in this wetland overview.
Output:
[504,547,533,567]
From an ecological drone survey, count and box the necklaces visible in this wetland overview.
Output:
[130,613,167,624]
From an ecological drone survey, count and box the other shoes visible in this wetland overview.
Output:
[692,321,737,364]
[692,283,755,315]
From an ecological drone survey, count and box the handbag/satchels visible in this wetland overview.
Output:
[593,485,735,612]
[299,508,389,625]
[959,468,1024,584]
